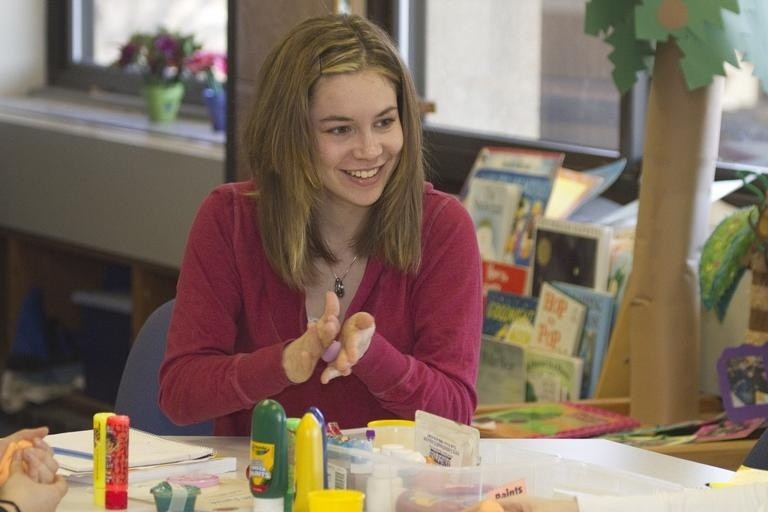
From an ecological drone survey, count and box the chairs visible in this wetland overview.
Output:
[111,295,217,436]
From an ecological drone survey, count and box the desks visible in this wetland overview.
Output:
[12,427,768,512]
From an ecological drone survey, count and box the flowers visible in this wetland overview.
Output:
[116,25,229,91]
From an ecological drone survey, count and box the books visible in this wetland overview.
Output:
[481,288,539,347]
[458,149,566,298]
[530,218,612,307]
[41,425,237,489]
[537,283,587,357]
[524,350,582,405]
[552,281,614,402]
[468,401,639,439]
[549,158,640,299]
[473,336,526,404]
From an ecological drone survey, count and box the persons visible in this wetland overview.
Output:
[0,425,69,512]
[465,476,767,512]
[157,15,484,435]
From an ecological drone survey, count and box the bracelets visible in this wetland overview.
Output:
[1,500,22,512]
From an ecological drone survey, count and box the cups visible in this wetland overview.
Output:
[367,419,416,449]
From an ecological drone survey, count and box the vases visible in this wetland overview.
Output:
[137,81,186,124]
[206,93,228,131]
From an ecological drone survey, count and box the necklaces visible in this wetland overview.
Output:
[327,244,358,298]
